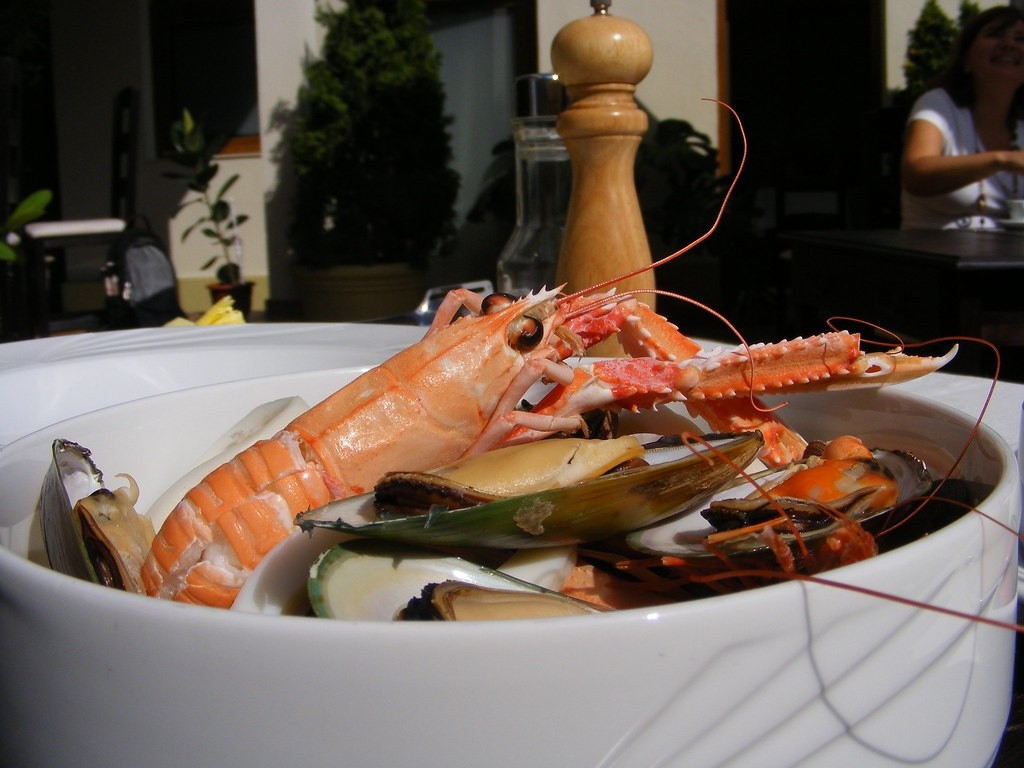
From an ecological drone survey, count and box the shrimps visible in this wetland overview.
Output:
[135,97,1024,635]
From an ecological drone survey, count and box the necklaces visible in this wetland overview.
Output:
[966,104,1019,222]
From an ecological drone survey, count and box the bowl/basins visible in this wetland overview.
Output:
[0,325,1024,768]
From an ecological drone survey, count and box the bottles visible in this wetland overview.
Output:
[496,73,573,298]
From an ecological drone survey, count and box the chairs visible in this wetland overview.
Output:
[23,86,138,337]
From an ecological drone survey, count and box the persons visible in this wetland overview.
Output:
[898,6,1024,229]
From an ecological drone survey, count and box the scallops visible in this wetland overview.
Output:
[31,392,931,623]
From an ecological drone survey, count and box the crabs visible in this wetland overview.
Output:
[700,434,898,574]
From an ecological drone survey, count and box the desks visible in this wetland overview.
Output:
[777,225,1024,270]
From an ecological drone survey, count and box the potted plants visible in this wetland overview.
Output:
[286,0,461,321]
[159,107,256,318]
